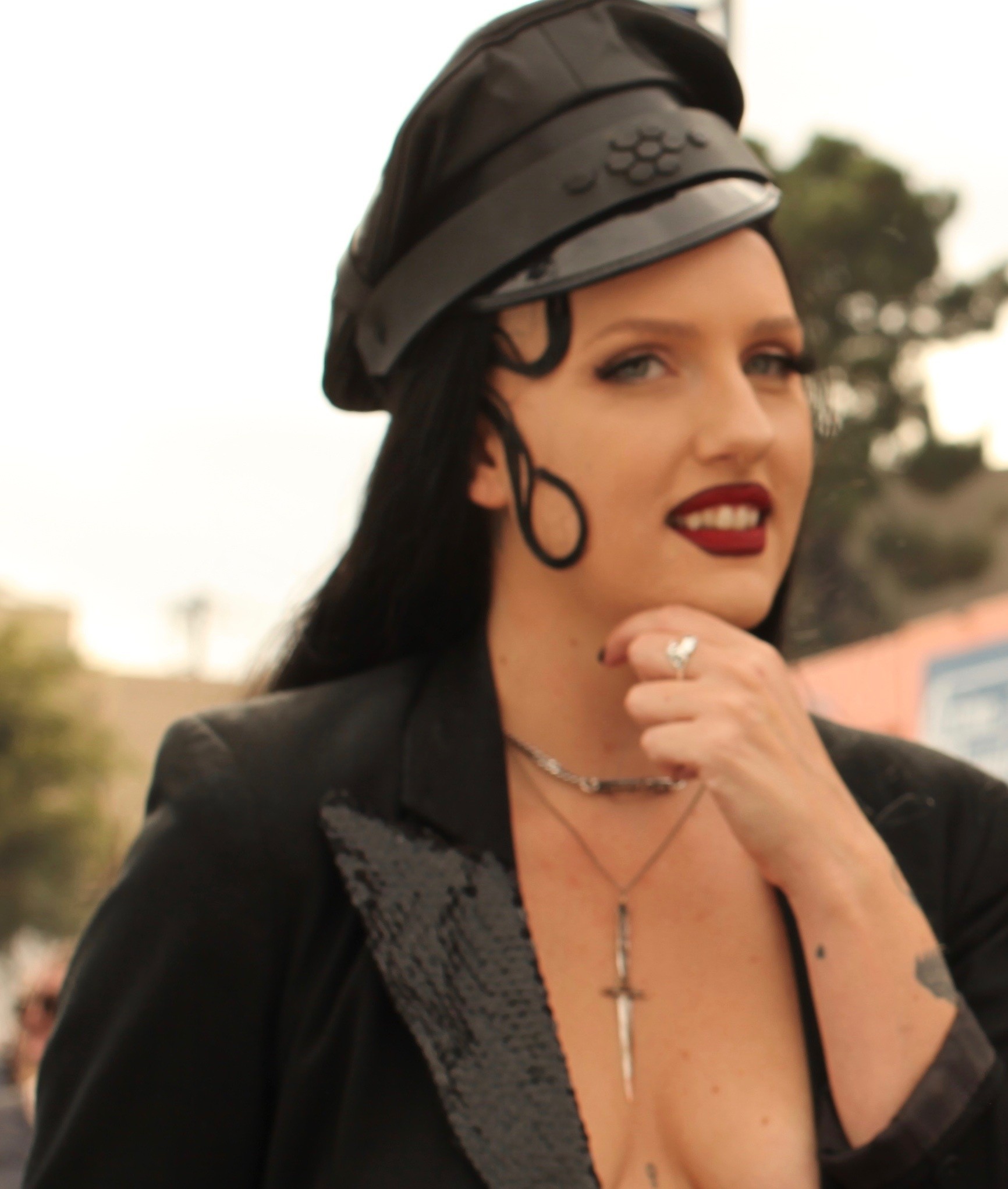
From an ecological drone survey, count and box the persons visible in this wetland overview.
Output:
[23,0,1007,1189]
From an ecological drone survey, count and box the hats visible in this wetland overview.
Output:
[324,0,785,410]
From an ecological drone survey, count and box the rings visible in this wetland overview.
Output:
[667,634,699,679]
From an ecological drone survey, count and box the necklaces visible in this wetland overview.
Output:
[509,745,710,1103]
[501,728,687,796]
